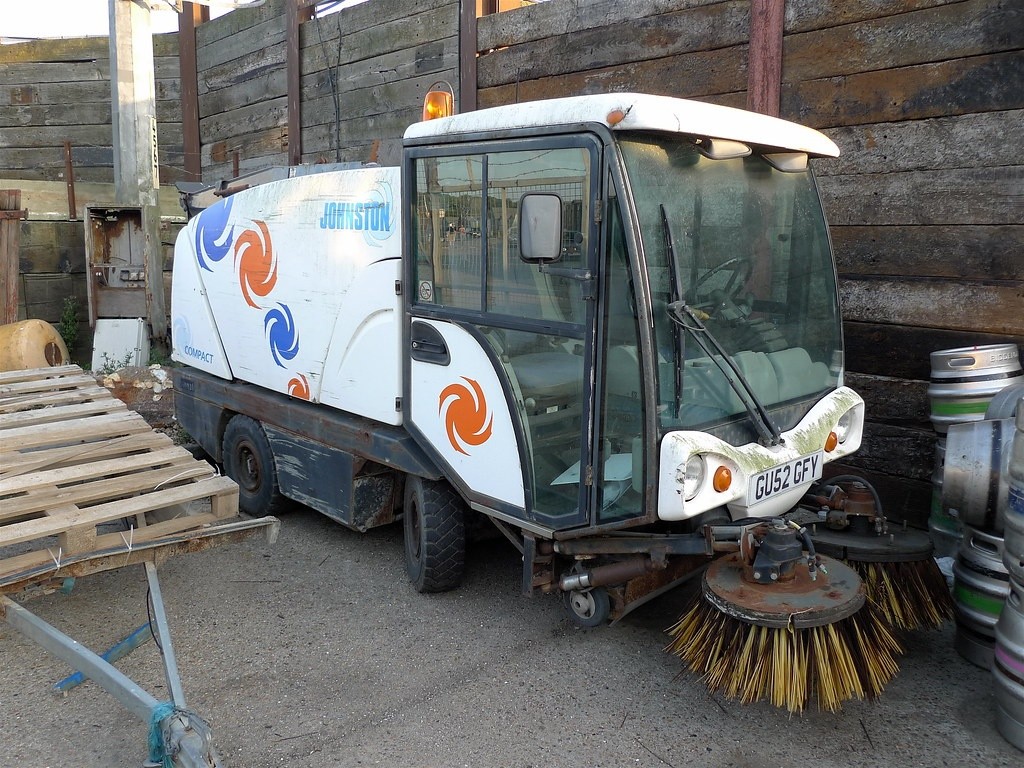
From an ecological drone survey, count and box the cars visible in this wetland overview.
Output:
[560,229,584,260]
[508,226,518,248]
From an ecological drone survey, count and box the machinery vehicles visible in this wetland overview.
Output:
[171,81,956,718]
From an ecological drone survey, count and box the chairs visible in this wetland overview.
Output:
[572,241,638,345]
[448,237,586,398]
